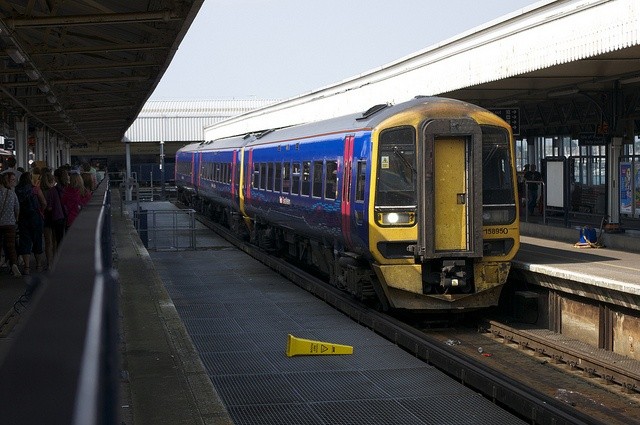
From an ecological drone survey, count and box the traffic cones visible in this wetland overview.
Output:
[287,333,354,358]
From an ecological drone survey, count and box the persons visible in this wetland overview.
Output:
[522,165,529,176]
[0,174,22,279]
[0,156,22,191]
[30,162,40,173]
[85,166,97,190]
[81,173,93,197]
[59,166,68,172]
[67,173,90,209]
[67,166,79,176]
[29,173,42,188]
[3,172,15,188]
[15,173,43,275]
[523,164,541,216]
[40,168,63,274]
[17,167,24,173]
[51,169,80,251]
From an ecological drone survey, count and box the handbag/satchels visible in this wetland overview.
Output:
[52,186,71,232]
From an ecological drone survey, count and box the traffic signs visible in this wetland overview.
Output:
[487,108,521,136]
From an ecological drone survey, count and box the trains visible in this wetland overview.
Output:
[174,95,520,329]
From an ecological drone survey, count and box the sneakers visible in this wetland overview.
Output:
[24,268,31,274]
[12,264,22,279]
